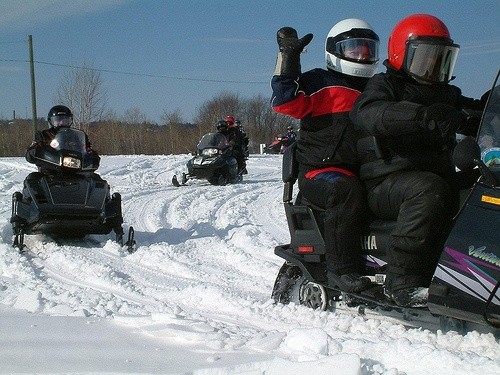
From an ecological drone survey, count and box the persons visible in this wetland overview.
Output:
[270,19,380,292]
[26,105,100,174]
[278,126,296,146]
[209,115,246,174]
[349,14,500,306]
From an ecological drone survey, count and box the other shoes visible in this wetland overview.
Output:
[383,286,434,308]
[326,270,370,292]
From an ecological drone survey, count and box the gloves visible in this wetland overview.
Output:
[274,27,314,78]
[422,103,465,140]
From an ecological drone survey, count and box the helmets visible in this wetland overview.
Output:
[47,105,73,129]
[382,13,451,70]
[287,125,293,129]
[216,120,228,128]
[325,18,380,79]
[234,120,240,123]
[225,116,235,124]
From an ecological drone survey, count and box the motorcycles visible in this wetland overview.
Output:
[263,134,296,154]
[11,128,136,254]
[271,68,500,331]
[172,132,248,186]
